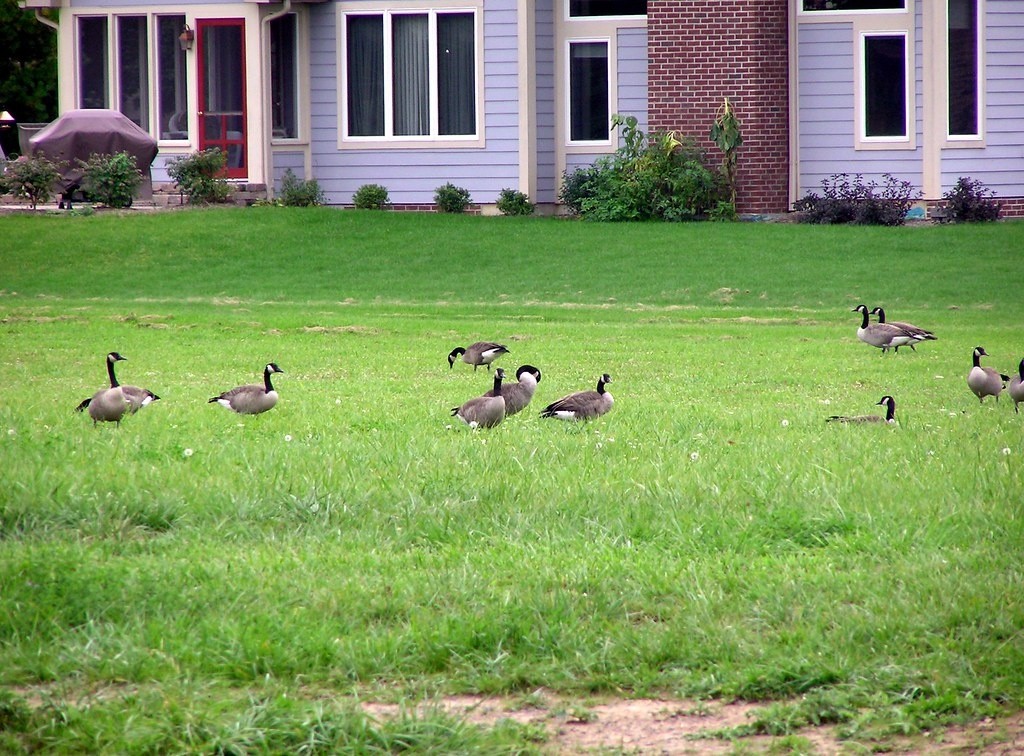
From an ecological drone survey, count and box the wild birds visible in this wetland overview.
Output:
[72,352,162,428]
[967,347,1024,417]
[851,305,938,356]
[450,364,541,432]
[448,341,510,373]
[826,396,895,426]
[539,373,615,423]
[207,362,284,414]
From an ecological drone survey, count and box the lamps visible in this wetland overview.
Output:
[177,23,193,50]
